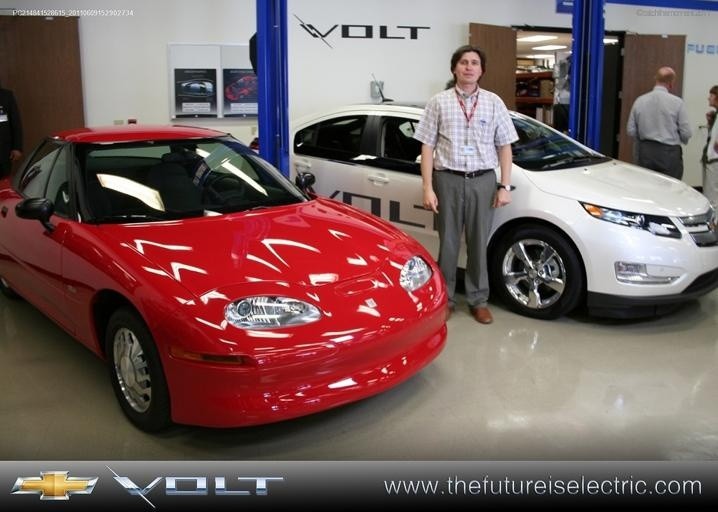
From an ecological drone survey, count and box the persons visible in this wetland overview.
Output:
[625,66,693,180]
[700,85,718,213]
[411,44,521,325]
[0,87,24,180]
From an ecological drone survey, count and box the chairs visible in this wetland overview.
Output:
[145,152,210,207]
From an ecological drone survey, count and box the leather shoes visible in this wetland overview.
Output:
[470,306,492,323]
[446,306,455,320]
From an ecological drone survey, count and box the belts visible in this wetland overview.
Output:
[447,169,492,178]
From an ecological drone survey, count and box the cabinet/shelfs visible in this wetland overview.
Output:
[516,72,555,128]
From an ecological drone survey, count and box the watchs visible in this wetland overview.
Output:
[498,185,511,191]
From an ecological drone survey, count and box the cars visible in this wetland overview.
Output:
[225,75,257,101]
[1,121,449,435]
[175,73,215,105]
[241,73,717,320]
